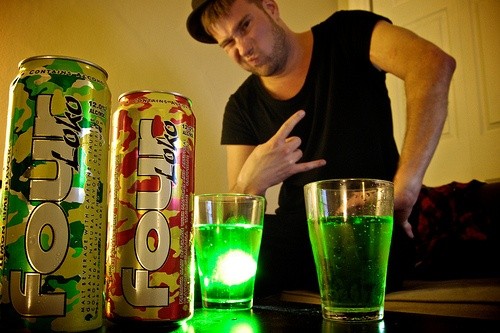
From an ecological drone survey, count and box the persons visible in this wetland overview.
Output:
[186,0,457,307]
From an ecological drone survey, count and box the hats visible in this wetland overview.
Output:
[185,0,217,45]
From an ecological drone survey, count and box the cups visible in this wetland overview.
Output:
[303,178,394,323]
[192,192,267,313]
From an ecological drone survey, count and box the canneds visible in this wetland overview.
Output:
[106,88,196,324]
[0,53,111,332]
